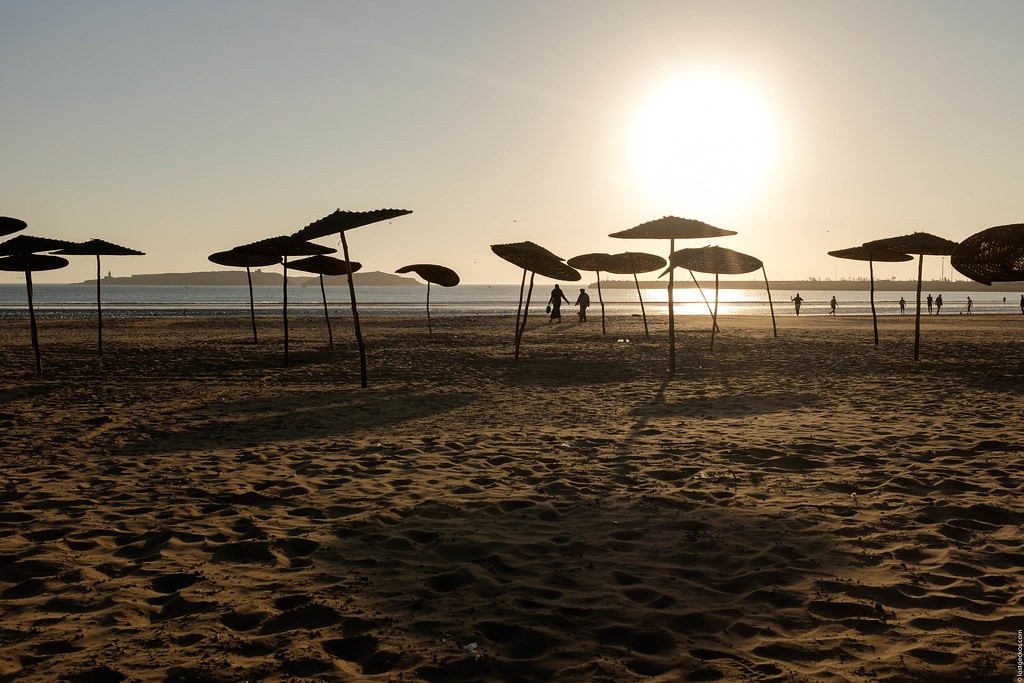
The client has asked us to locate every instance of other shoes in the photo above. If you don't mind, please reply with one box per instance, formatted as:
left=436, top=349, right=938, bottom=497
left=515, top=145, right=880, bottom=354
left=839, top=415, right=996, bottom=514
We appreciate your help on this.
left=583, top=321, right=586, bottom=322
left=548, top=320, right=551, bottom=322
left=557, top=321, right=561, bottom=323
left=578, top=319, right=581, bottom=322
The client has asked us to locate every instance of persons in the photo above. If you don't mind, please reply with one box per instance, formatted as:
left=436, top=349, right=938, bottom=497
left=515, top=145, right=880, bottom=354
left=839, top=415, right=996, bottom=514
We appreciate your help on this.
left=927, top=294, right=934, bottom=314
left=966, top=296, right=973, bottom=314
left=935, top=294, right=943, bottom=314
left=1020, top=295, right=1024, bottom=314
left=791, top=294, right=804, bottom=315
left=829, top=296, right=839, bottom=316
left=548, top=284, right=570, bottom=322
left=575, top=289, right=590, bottom=322
left=899, top=297, right=906, bottom=315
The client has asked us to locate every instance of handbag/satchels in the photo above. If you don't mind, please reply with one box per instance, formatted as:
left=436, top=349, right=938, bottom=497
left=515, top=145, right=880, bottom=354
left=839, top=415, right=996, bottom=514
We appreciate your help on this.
left=546, top=305, right=550, bottom=313
